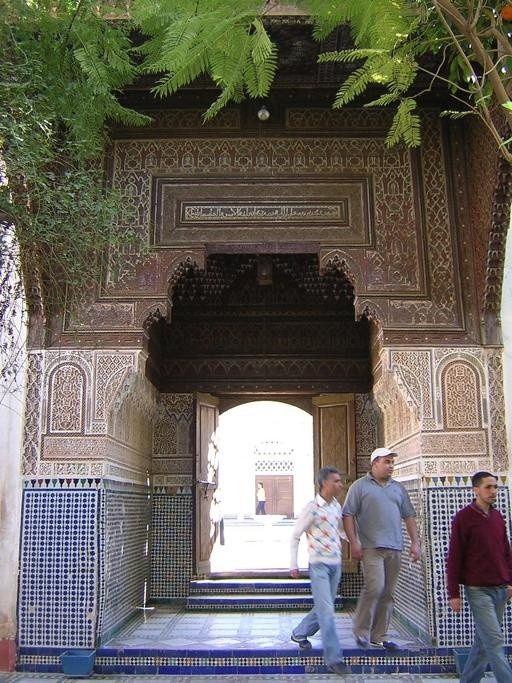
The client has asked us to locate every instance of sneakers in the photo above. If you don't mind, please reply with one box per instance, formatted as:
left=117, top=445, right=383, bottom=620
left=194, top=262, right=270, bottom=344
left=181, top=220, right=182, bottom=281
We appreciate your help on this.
left=326, top=661, right=352, bottom=674
left=368, top=638, right=400, bottom=651
left=352, top=629, right=371, bottom=649
left=290, top=634, right=312, bottom=649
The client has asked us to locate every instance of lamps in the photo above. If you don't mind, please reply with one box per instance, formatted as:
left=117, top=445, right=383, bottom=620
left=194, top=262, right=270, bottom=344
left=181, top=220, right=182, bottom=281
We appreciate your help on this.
left=256, top=253, right=274, bottom=285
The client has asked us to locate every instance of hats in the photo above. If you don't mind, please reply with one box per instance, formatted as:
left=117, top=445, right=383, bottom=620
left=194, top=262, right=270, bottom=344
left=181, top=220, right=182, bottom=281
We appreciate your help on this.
left=370, top=447, right=399, bottom=465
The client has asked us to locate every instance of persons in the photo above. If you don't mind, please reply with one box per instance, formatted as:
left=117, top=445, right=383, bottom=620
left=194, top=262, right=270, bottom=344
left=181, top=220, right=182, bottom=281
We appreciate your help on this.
left=342, top=446, right=422, bottom=649
left=445, top=470, right=512, bottom=683
left=254, top=481, right=268, bottom=514
left=209, top=484, right=226, bottom=547
left=289, top=465, right=362, bottom=678
